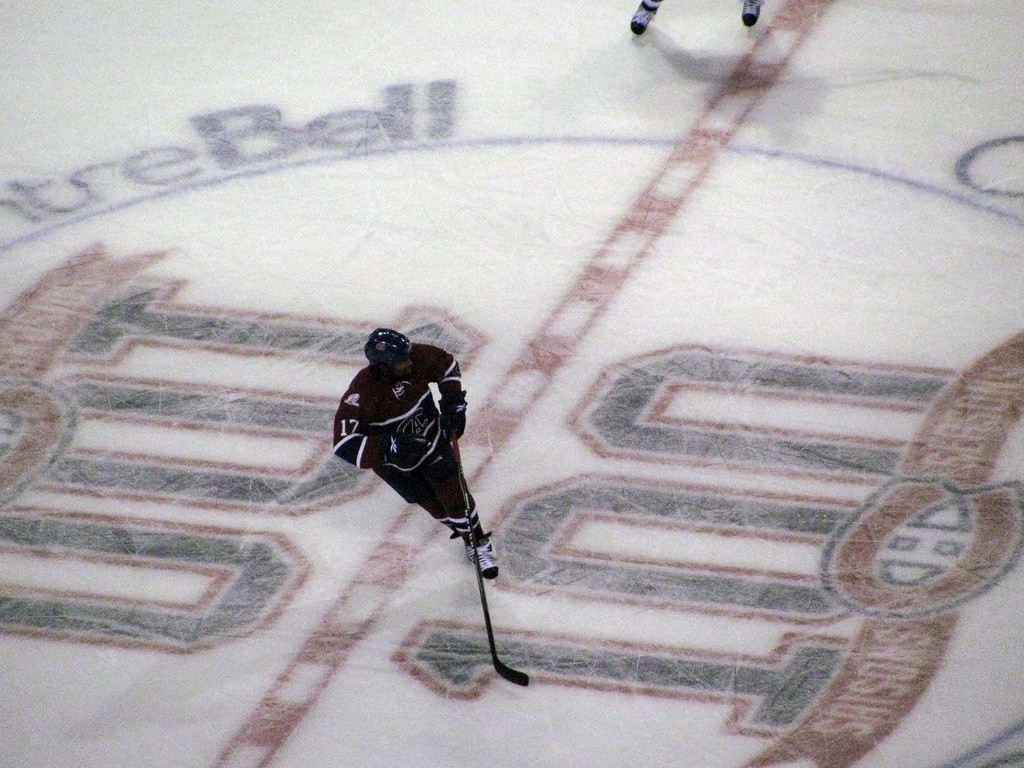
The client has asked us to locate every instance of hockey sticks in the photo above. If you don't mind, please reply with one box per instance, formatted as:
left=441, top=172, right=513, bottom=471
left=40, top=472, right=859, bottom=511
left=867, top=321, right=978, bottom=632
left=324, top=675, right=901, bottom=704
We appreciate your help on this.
left=447, top=431, right=531, bottom=688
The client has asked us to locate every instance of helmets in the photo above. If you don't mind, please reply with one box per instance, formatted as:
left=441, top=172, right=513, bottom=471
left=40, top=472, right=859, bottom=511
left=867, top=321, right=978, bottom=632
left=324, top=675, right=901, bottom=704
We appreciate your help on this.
left=364, top=328, right=410, bottom=371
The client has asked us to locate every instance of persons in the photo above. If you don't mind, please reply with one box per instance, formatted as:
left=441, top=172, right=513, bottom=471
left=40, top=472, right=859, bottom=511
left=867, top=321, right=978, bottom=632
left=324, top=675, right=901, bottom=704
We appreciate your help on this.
left=631, top=0, right=761, bottom=40
left=333, top=328, right=498, bottom=586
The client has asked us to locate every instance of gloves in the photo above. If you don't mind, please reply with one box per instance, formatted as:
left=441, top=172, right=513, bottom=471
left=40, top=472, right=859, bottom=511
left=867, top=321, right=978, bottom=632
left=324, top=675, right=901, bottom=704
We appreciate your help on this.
left=440, top=391, right=466, bottom=439
left=377, top=434, right=432, bottom=470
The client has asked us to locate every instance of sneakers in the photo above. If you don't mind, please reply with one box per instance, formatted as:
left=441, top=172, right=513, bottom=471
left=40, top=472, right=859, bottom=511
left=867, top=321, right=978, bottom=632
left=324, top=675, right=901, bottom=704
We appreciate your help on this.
left=630, top=0, right=663, bottom=35
left=742, top=0, right=764, bottom=27
left=465, top=531, right=498, bottom=579
